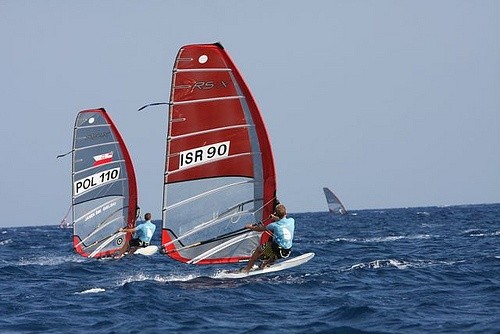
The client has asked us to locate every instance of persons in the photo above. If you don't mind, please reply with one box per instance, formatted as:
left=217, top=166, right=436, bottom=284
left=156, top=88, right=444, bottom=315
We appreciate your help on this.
left=118, top=212, right=156, bottom=253
left=240, top=204, right=295, bottom=272
left=338, top=207, right=343, bottom=215
